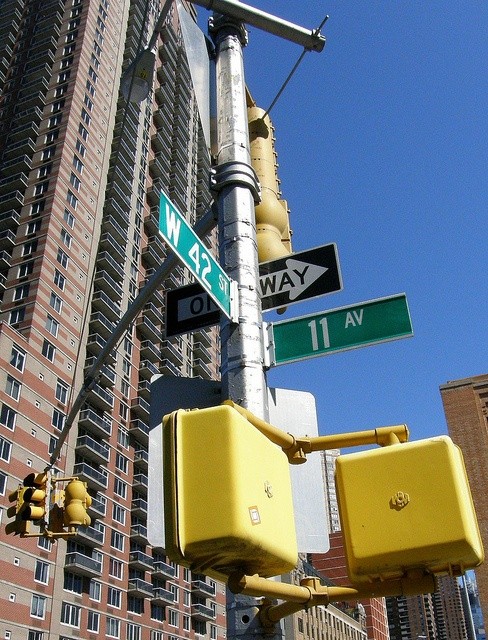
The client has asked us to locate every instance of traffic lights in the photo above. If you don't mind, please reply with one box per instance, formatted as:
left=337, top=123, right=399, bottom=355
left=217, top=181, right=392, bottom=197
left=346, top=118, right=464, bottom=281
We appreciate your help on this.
left=22, top=473, right=48, bottom=522
left=6, top=483, right=34, bottom=540
left=65, top=481, right=93, bottom=528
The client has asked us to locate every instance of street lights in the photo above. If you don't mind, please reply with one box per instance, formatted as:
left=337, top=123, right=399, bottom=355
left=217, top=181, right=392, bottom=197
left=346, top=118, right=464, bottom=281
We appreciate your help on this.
left=118, top=1, right=328, bottom=640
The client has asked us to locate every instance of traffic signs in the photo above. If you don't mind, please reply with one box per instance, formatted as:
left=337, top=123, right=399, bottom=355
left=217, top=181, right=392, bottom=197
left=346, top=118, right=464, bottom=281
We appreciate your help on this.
left=165, top=241, right=342, bottom=346
left=262, top=285, right=415, bottom=375
left=159, top=179, right=245, bottom=326
